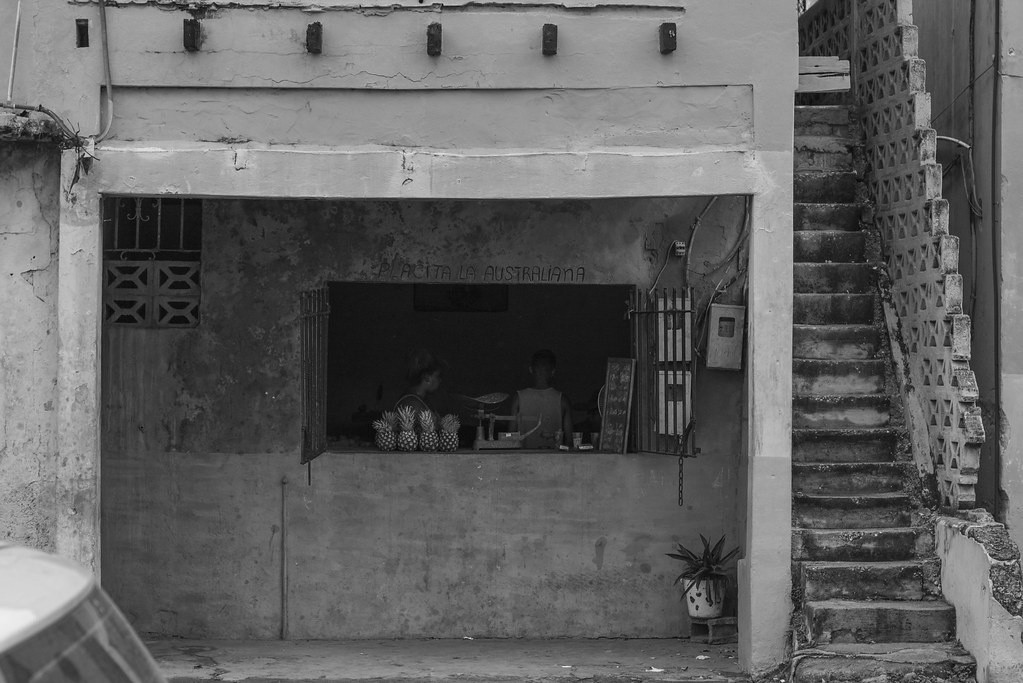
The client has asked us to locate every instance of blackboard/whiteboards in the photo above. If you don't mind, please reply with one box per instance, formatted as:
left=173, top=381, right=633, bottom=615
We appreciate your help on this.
left=599, top=357, right=636, bottom=453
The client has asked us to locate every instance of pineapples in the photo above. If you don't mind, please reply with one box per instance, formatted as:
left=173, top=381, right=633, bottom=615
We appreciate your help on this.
left=370, top=405, right=461, bottom=452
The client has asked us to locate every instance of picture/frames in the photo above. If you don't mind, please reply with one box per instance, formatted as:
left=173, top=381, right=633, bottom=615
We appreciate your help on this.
left=413, top=284, right=508, bottom=313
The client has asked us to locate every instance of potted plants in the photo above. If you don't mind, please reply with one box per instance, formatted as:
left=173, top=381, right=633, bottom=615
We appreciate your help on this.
left=662, top=531, right=741, bottom=620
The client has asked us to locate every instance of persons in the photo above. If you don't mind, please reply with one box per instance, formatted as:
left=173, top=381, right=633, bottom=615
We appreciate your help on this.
left=508, top=349, right=573, bottom=449
left=397, top=345, right=444, bottom=430
